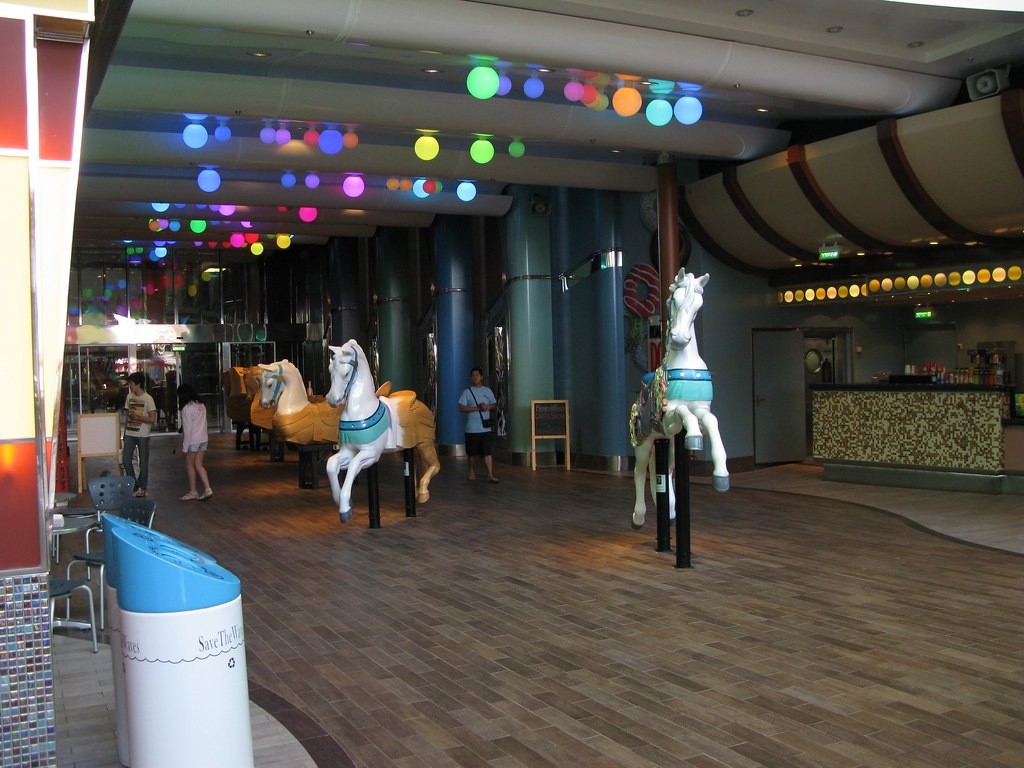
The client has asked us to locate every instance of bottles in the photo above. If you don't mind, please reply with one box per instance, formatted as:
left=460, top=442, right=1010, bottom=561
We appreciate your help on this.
left=904, top=361, right=931, bottom=375
left=932, top=362, right=973, bottom=384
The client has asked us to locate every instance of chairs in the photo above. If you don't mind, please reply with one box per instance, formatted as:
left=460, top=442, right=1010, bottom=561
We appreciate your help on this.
left=49, top=473, right=158, bottom=654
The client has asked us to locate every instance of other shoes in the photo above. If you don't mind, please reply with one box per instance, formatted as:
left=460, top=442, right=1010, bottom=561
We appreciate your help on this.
left=489, top=477, right=499, bottom=483
left=197, top=488, right=213, bottom=501
left=469, top=475, right=476, bottom=481
left=136, top=487, right=146, bottom=496
left=179, top=491, right=200, bottom=501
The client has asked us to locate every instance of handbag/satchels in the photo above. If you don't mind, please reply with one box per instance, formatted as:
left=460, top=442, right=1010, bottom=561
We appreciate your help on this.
left=482, top=418, right=495, bottom=428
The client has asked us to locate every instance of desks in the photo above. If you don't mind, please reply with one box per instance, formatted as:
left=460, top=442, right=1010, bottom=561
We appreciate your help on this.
left=54, top=491, right=77, bottom=508
left=51, top=517, right=95, bottom=632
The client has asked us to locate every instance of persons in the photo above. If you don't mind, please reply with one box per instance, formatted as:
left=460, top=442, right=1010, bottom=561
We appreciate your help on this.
left=177, top=383, right=213, bottom=501
left=122, top=372, right=157, bottom=496
left=458, top=368, right=500, bottom=483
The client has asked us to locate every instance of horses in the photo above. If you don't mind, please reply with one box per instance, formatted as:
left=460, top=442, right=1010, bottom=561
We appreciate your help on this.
left=239, top=366, right=276, bottom=429
left=629, top=267, right=729, bottom=531
left=258, top=359, right=339, bottom=444
left=325, top=339, right=441, bottom=524
